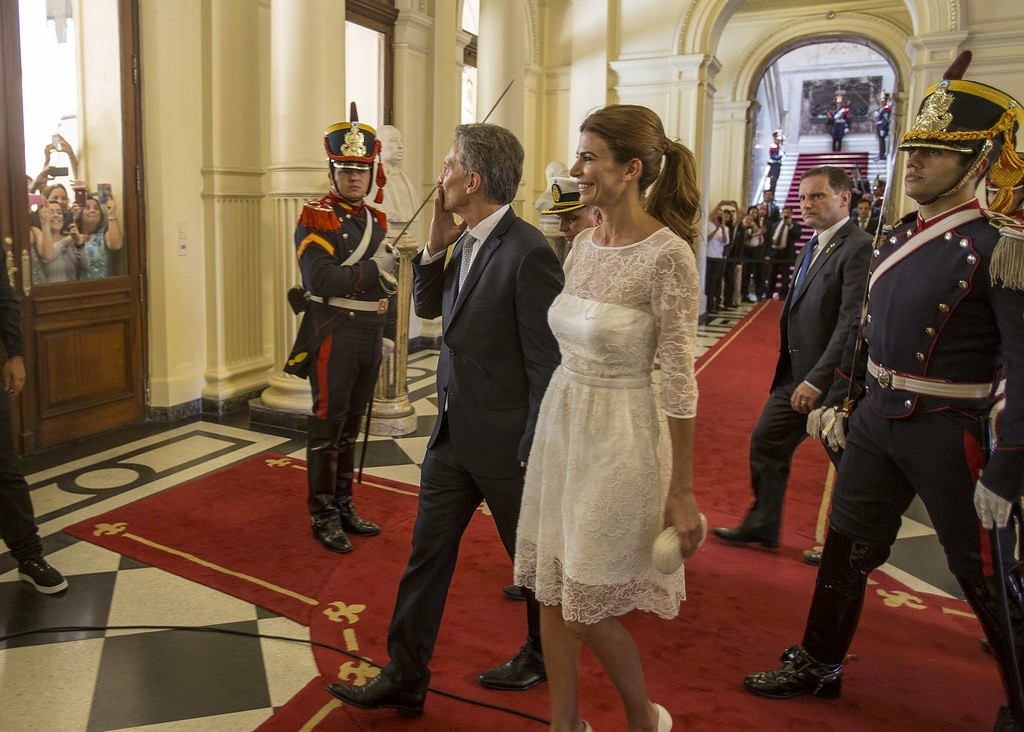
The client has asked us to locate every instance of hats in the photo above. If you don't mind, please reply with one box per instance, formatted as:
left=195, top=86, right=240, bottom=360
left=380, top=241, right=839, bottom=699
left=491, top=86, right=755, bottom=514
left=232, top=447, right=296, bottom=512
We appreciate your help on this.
left=899, top=50, right=1023, bottom=171
left=540, top=178, right=586, bottom=215
left=324, top=121, right=387, bottom=203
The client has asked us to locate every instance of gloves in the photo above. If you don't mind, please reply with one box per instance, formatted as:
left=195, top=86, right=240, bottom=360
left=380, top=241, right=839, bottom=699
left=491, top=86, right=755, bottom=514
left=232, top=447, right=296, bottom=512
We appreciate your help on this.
left=972, top=480, right=1012, bottom=528
left=373, top=238, right=401, bottom=276
left=821, top=408, right=847, bottom=452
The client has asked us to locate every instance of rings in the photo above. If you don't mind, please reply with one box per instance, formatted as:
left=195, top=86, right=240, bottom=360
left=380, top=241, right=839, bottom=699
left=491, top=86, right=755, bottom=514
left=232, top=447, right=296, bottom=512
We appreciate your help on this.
left=801, top=400, right=807, bottom=405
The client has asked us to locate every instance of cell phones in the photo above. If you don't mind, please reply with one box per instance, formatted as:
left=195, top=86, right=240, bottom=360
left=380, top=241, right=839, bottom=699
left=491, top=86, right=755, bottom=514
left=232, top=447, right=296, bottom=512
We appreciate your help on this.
left=62, top=212, right=73, bottom=229
left=74, top=187, right=86, bottom=208
left=52, top=135, right=58, bottom=147
left=97, top=184, right=113, bottom=204
left=50, top=167, right=68, bottom=176
left=27, top=195, right=46, bottom=205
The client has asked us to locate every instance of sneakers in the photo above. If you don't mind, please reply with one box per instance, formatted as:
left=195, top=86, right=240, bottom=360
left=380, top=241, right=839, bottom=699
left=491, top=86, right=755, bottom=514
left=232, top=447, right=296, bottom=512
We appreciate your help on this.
left=17, top=556, right=68, bottom=595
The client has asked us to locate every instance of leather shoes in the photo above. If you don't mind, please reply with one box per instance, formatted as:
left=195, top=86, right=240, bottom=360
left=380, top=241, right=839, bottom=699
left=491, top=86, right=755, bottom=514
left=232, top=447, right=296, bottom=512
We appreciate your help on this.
left=712, top=527, right=778, bottom=548
left=744, top=646, right=843, bottom=699
left=477, top=647, right=546, bottom=691
left=324, top=673, right=425, bottom=718
left=803, top=552, right=820, bottom=564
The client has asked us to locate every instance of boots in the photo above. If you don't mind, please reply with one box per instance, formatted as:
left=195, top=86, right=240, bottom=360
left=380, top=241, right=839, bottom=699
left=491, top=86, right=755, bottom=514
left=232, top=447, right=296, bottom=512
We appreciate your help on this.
left=336, top=414, right=382, bottom=536
left=307, top=414, right=352, bottom=553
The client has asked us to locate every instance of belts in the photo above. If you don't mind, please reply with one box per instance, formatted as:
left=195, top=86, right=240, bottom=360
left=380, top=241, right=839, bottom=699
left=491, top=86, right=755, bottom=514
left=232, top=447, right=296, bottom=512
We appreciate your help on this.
left=310, top=295, right=389, bottom=315
left=866, top=357, right=993, bottom=398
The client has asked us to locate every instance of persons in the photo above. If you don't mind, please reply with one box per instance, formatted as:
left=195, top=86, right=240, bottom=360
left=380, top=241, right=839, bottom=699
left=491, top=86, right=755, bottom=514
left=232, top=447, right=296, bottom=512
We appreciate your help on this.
left=711, top=165, right=875, bottom=567
left=282, top=101, right=399, bottom=551
left=534, top=160, right=570, bottom=211
left=365, top=124, right=422, bottom=218
left=765, top=128, right=786, bottom=203
left=513, top=105, right=704, bottom=732
left=325, top=122, right=567, bottom=720
left=0, top=238, right=70, bottom=593
left=503, top=176, right=604, bottom=601
left=704, top=189, right=801, bottom=316
left=25, top=135, right=123, bottom=285
left=735, top=52, right=1023, bottom=732
left=874, top=92, right=891, bottom=159
left=824, top=95, right=851, bottom=152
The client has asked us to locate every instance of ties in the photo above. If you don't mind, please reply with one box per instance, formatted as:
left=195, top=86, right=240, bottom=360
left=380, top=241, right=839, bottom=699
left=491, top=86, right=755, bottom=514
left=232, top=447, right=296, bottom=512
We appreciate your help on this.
left=861, top=220, right=866, bottom=230
left=776, top=223, right=786, bottom=245
left=458, top=234, right=477, bottom=293
left=790, top=236, right=819, bottom=308
left=766, top=205, right=769, bottom=217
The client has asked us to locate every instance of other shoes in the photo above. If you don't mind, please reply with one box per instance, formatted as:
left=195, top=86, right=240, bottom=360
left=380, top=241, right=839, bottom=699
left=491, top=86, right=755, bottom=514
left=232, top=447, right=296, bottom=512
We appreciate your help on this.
left=650, top=701, right=672, bottom=731
left=981, top=638, right=995, bottom=655
left=993, top=706, right=1023, bottom=732
left=581, top=719, right=593, bottom=732
left=504, top=584, right=527, bottom=601
left=706, top=285, right=788, bottom=314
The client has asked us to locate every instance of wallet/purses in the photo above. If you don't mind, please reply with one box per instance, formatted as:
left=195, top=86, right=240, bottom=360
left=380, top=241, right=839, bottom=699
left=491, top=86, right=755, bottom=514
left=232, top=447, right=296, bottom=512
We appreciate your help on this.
left=652, top=511, right=708, bottom=575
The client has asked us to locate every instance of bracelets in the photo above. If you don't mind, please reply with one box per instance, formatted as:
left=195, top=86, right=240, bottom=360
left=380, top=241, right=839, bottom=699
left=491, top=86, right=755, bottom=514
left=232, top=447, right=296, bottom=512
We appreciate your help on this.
left=75, top=240, right=84, bottom=248
left=108, top=217, right=118, bottom=221
